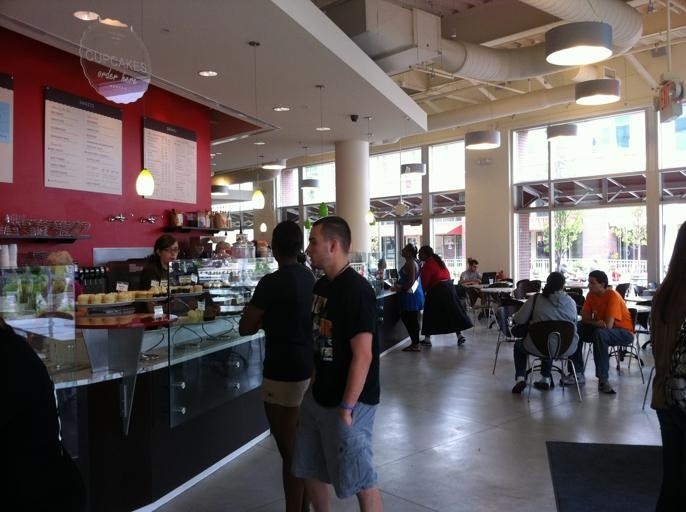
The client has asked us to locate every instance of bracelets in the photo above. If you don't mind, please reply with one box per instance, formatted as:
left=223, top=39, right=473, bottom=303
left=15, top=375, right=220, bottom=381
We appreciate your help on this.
left=338, top=402, right=357, bottom=412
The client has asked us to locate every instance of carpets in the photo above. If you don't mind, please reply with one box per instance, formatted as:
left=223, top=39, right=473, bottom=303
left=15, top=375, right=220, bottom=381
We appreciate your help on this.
left=546, top=440, right=665, bottom=511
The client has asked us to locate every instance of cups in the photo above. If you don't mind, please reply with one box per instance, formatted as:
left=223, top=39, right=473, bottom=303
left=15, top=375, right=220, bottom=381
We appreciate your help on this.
left=1, top=244, right=18, bottom=269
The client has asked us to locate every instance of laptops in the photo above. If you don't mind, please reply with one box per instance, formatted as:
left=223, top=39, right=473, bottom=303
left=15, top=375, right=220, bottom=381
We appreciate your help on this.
left=479, top=272, right=496, bottom=284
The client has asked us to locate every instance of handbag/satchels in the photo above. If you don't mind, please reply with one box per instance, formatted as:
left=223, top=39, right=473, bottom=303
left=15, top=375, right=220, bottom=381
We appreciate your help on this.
left=393, top=278, right=419, bottom=294
left=511, top=324, right=528, bottom=338
left=663, top=324, right=686, bottom=414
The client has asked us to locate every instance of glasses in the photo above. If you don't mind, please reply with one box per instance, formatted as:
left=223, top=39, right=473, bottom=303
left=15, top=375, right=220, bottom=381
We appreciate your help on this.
left=162, top=247, right=180, bottom=253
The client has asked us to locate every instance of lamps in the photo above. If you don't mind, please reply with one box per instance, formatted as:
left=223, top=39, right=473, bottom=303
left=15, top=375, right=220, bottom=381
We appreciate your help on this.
left=211, top=185, right=229, bottom=196
left=248, top=40, right=265, bottom=209
left=575, top=79, right=620, bottom=107
left=136, top=1, right=155, bottom=196
left=465, top=130, right=500, bottom=150
left=305, top=219, right=310, bottom=229
left=547, top=123, right=578, bottom=141
left=315, top=85, right=328, bottom=219
left=300, top=179, right=320, bottom=191
left=545, top=22, right=613, bottom=66
left=401, top=163, right=426, bottom=176
left=394, top=138, right=409, bottom=216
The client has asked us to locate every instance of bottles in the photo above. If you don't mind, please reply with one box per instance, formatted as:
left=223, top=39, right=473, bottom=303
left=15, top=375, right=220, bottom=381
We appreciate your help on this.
left=74, top=262, right=112, bottom=294
left=188, top=211, right=232, bottom=228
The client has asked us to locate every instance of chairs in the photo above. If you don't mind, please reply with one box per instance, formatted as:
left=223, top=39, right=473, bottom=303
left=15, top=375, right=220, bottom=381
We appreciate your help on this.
left=461, top=278, right=657, bottom=412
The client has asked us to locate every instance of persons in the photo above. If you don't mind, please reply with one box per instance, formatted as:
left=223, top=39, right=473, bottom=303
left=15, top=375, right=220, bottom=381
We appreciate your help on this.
left=1, top=317, right=59, bottom=511
left=460, top=259, right=486, bottom=308
left=513, top=271, right=579, bottom=393
left=391, top=244, right=425, bottom=352
left=135, top=234, right=180, bottom=313
left=239, top=221, right=314, bottom=511
left=651, top=220, right=685, bottom=511
left=27, top=250, right=86, bottom=347
left=419, top=245, right=473, bottom=346
left=293, top=217, right=384, bottom=511
left=561, top=271, right=634, bottom=394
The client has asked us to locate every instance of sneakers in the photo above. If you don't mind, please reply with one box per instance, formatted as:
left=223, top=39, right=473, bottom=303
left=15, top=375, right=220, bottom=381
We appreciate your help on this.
left=420, top=340, right=432, bottom=345
left=458, top=337, right=465, bottom=345
left=559, top=372, right=585, bottom=384
left=534, top=381, right=550, bottom=390
left=402, top=344, right=422, bottom=351
left=512, top=381, right=527, bottom=393
left=598, top=382, right=616, bottom=394
